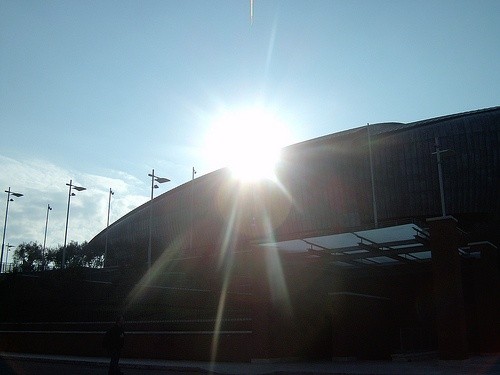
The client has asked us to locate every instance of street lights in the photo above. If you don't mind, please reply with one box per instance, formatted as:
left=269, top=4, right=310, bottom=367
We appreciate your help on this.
left=191, top=167, right=197, bottom=258
left=5, top=243, right=15, bottom=273
left=42, top=204, right=53, bottom=263
left=63, top=179, right=86, bottom=269
left=147, top=169, right=171, bottom=271
left=0, top=186, right=24, bottom=273
left=105, top=188, right=115, bottom=266
